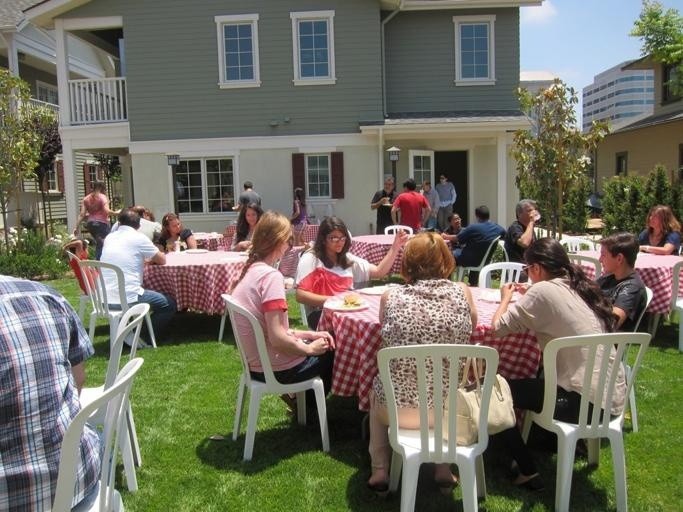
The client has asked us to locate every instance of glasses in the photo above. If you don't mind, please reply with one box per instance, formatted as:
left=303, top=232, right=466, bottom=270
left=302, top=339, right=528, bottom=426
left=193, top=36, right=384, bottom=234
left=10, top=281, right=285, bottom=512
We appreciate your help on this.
left=329, top=236, right=347, bottom=242
left=522, top=264, right=535, bottom=277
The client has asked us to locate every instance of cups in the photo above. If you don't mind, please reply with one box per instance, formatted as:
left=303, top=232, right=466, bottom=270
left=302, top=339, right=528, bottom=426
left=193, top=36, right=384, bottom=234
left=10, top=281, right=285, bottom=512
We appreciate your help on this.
left=384, top=197, right=390, bottom=204
left=420, top=190, right=425, bottom=195
left=531, top=210, right=540, bottom=221
left=174, top=241, right=180, bottom=254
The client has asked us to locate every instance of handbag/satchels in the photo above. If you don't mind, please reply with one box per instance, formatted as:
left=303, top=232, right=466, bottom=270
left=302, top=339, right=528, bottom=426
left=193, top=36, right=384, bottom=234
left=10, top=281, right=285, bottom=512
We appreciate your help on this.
left=441, top=374, right=517, bottom=446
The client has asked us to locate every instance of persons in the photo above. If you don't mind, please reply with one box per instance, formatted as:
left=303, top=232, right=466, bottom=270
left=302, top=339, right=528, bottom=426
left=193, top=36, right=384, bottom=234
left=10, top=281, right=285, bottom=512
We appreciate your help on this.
left=370, top=173, right=540, bottom=284
left=288, top=187, right=310, bottom=224
left=592, top=229, right=647, bottom=334
left=96, top=211, right=176, bottom=349
left=226, top=210, right=337, bottom=421
left=491, top=236, right=627, bottom=493
left=293, top=215, right=409, bottom=340
left=73, top=179, right=122, bottom=261
left=0, top=274, right=115, bottom=511
left=232, top=182, right=261, bottom=211
left=112, top=203, right=197, bottom=254
left=230, top=201, right=265, bottom=252
left=637, top=205, right=682, bottom=336
left=367, top=231, right=477, bottom=498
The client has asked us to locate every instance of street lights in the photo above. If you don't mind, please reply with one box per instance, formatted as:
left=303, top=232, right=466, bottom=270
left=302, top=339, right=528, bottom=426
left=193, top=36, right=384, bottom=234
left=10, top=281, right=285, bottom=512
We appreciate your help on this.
left=164, top=151, right=181, bottom=219
left=386, top=146, right=401, bottom=223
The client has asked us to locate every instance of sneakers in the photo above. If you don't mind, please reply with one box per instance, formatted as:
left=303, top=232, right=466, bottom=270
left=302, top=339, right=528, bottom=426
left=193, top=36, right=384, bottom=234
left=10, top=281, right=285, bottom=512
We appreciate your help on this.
left=516, top=472, right=547, bottom=493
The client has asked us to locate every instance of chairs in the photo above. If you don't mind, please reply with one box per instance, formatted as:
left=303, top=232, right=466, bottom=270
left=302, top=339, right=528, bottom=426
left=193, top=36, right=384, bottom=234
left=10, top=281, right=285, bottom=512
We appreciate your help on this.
left=69, top=301, right=152, bottom=493
left=369, top=338, right=501, bottom=510
left=517, top=329, right=654, bottom=507
left=43, top=355, right=128, bottom=511
left=217, top=291, right=331, bottom=463
left=24, top=214, right=679, bottom=353
left=620, top=283, right=652, bottom=436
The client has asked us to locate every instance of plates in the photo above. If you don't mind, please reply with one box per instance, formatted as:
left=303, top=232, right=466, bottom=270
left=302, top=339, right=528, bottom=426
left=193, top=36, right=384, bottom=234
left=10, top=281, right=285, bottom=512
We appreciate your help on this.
left=382, top=204, right=393, bottom=206
left=321, top=299, right=370, bottom=311
left=478, top=290, right=518, bottom=303
left=360, top=285, right=388, bottom=296
left=185, top=248, right=208, bottom=253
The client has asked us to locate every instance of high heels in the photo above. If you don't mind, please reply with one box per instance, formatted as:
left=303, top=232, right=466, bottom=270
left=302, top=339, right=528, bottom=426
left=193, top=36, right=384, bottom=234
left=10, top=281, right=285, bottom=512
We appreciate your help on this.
left=437, top=472, right=458, bottom=496
left=367, top=479, right=390, bottom=498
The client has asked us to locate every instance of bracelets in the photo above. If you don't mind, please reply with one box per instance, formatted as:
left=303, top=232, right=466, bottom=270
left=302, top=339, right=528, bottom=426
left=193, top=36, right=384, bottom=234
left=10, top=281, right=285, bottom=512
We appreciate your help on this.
left=646, top=244, right=650, bottom=252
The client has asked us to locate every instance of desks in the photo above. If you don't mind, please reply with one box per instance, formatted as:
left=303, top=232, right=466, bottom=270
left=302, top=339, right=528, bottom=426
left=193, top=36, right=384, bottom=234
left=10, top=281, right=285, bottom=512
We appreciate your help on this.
left=320, top=276, right=542, bottom=453
